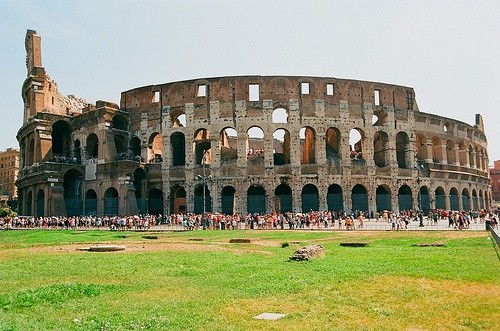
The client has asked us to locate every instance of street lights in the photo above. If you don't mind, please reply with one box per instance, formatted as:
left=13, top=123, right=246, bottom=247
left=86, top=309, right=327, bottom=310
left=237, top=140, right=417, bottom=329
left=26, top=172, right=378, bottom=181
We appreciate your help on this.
left=418, top=164, right=424, bottom=215
left=202, top=149, right=211, bottom=217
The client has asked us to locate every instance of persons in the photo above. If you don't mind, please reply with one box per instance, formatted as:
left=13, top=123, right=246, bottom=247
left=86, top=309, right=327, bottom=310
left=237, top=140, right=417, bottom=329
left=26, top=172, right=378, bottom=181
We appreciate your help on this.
left=0, top=149, right=498, bottom=235
